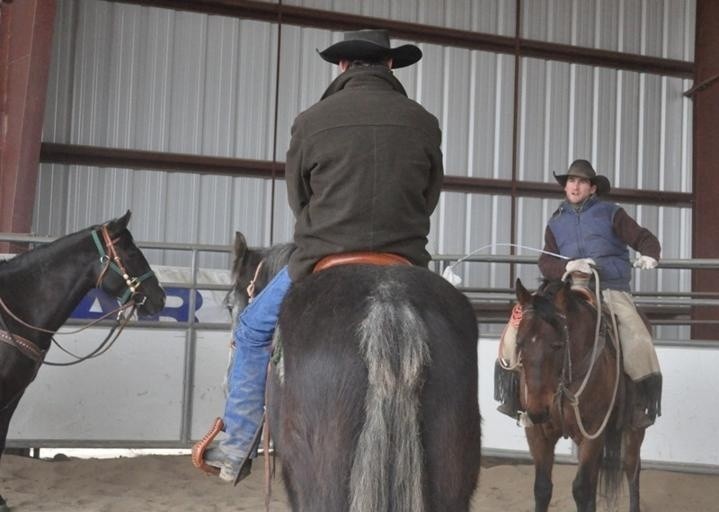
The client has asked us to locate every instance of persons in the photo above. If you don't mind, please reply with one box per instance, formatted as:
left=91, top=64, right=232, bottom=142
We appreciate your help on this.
left=493, top=158, right=661, bottom=426
left=197, top=28, right=444, bottom=484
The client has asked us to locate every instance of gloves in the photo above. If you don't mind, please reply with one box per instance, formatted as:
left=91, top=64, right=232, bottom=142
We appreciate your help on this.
left=634, top=256, right=657, bottom=271
left=566, top=258, right=594, bottom=277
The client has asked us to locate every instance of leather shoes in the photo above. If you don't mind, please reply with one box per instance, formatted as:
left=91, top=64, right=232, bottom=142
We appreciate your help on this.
left=633, top=413, right=652, bottom=431
left=203, top=447, right=250, bottom=483
left=497, top=403, right=514, bottom=414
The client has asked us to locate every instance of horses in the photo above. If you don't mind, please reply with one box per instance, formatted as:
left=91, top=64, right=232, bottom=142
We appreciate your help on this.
left=220, top=229, right=484, bottom=512
left=1, top=206, right=168, bottom=512
left=513, top=277, right=646, bottom=512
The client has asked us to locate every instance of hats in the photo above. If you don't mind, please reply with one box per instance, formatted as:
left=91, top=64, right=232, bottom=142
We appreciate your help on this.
left=316, top=30, right=422, bottom=69
left=553, top=160, right=610, bottom=195
